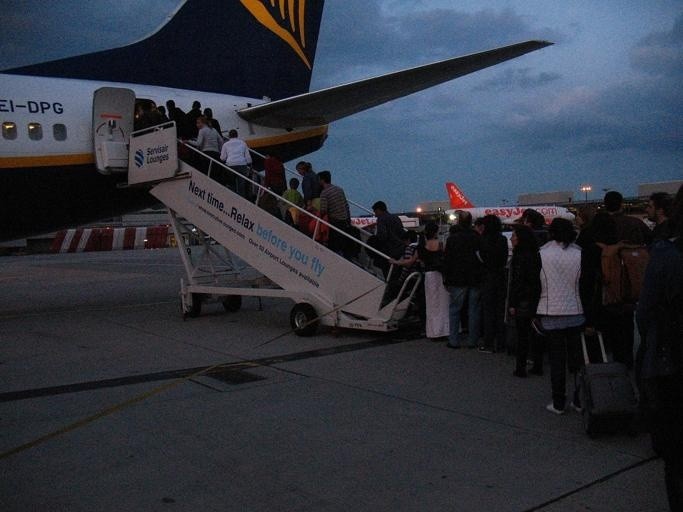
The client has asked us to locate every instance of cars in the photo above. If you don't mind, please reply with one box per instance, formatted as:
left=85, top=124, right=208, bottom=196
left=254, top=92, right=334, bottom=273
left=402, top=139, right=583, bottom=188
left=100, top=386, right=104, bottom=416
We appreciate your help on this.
left=173, top=216, right=199, bottom=241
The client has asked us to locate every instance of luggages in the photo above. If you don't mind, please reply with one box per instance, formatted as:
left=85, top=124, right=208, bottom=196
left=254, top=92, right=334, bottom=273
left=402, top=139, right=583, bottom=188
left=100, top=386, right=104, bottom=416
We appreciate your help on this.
left=580, top=331, right=640, bottom=430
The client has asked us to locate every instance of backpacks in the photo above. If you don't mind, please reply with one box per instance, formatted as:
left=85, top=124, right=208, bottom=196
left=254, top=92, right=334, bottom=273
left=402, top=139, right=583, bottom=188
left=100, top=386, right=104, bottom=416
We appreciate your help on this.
left=596, top=239, right=648, bottom=306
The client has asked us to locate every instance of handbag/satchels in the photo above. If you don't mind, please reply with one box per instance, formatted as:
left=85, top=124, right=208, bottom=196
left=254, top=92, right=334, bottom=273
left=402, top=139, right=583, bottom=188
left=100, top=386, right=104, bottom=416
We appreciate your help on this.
left=349, top=226, right=361, bottom=252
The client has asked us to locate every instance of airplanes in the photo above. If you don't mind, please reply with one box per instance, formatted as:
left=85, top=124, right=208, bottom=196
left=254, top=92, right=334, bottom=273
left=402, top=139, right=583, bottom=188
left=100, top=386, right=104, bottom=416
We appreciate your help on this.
left=441, top=181, right=579, bottom=229
left=0, top=1, right=555, bottom=244
left=351, top=214, right=420, bottom=232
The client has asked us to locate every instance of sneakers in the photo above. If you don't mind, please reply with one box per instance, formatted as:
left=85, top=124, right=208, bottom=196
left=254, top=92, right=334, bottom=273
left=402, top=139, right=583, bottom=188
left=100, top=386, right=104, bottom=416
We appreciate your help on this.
left=545, top=404, right=563, bottom=414
left=528, top=368, right=544, bottom=376
left=477, top=344, right=494, bottom=351
left=513, top=368, right=526, bottom=377
left=570, top=401, right=583, bottom=412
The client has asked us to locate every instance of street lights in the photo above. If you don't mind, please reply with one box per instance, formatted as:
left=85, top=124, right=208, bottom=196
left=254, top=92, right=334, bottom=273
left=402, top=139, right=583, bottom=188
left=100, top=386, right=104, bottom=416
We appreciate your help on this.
left=582, top=185, right=591, bottom=201
